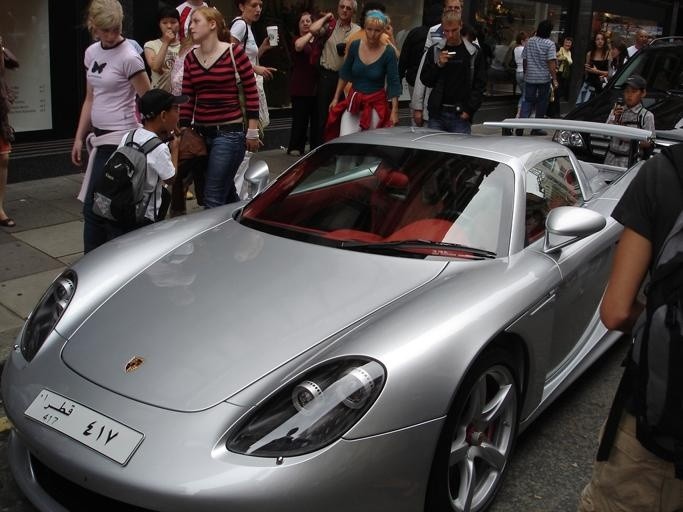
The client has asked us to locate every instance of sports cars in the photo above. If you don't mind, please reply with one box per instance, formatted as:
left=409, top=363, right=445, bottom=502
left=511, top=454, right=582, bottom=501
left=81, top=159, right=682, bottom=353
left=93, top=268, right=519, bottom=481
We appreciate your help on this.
left=1, top=118, right=682, bottom=511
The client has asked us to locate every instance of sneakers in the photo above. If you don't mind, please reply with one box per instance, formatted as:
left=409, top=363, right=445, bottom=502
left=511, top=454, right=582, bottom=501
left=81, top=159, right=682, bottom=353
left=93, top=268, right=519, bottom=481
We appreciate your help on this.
left=290, top=150, right=301, bottom=157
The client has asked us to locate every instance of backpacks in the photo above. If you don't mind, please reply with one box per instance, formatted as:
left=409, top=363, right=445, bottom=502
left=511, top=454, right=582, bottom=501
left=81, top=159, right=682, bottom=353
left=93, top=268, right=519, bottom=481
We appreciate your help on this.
left=88, top=128, right=165, bottom=230
left=595, top=209, right=683, bottom=482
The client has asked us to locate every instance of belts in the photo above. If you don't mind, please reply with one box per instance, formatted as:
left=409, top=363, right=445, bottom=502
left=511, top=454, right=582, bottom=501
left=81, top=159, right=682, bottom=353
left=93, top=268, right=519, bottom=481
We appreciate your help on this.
left=195, top=123, right=242, bottom=133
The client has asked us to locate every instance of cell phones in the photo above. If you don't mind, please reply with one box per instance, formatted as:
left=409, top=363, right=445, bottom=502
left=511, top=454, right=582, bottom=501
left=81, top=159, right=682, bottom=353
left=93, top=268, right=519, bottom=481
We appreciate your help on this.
left=617, top=94, right=625, bottom=111
left=448, top=52, right=456, bottom=55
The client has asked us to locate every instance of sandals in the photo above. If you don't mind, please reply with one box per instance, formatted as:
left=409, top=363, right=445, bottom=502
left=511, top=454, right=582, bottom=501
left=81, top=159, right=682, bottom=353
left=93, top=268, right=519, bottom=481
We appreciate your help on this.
left=1, top=216, right=14, bottom=227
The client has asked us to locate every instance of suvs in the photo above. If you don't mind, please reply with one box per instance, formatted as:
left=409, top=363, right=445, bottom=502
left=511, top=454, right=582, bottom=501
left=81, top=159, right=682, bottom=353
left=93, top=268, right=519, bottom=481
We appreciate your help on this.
left=551, top=35, right=682, bottom=164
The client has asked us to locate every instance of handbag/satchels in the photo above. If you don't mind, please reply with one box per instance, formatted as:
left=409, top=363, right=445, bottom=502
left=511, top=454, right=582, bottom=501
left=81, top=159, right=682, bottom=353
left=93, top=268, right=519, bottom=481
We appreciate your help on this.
left=310, top=18, right=337, bottom=68
left=230, top=43, right=264, bottom=143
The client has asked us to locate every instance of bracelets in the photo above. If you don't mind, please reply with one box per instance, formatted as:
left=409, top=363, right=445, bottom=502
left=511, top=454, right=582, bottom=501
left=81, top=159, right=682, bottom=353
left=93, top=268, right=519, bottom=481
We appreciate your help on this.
left=1, top=46, right=4, bottom=52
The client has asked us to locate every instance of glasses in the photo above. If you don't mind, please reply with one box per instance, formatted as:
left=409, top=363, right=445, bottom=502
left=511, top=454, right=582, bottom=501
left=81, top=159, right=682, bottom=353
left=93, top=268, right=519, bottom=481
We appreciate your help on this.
left=340, top=4, right=353, bottom=12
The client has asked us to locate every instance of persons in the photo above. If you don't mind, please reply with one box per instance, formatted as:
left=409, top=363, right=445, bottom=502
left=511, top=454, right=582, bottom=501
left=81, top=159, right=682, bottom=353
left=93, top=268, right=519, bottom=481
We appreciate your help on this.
left=556, top=36, right=572, bottom=79
left=397, top=11, right=440, bottom=127
left=71, top=0, right=152, bottom=255
left=513, top=31, right=529, bottom=118
left=175, top=0, right=209, bottom=45
left=227, top=0, right=279, bottom=202
left=489, top=34, right=510, bottom=95
left=308, top=0, right=361, bottom=151
left=120, top=20, right=152, bottom=84
left=576, top=143, right=683, bottom=512
left=142, top=7, right=193, bottom=219
left=323, top=9, right=402, bottom=176
left=177, top=6, right=260, bottom=211
left=0, top=36, right=20, bottom=227
left=574, top=30, right=609, bottom=109
left=602, top=74, right=655, bottom=168
left=419, top=10, right=488, bottom=134
left=408, top=23, right=477, bottom=128
left=601, top=41, right=629, bottom=80
left=514, top=20, right=559, bottom=136
left=624, top=29, right=647, bottom=64
left=423, top=0, right=480, bottom=52
left=115, top=88, right=181, bottom=236
left=287, top=11, right=317, bottom=155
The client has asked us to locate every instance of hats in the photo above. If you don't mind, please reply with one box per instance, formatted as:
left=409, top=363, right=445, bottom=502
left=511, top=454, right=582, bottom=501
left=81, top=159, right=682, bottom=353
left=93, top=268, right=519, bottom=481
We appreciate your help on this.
left=621, top=75, right=647, bottom=91
left=138, top=89, right=189, bottom=120
